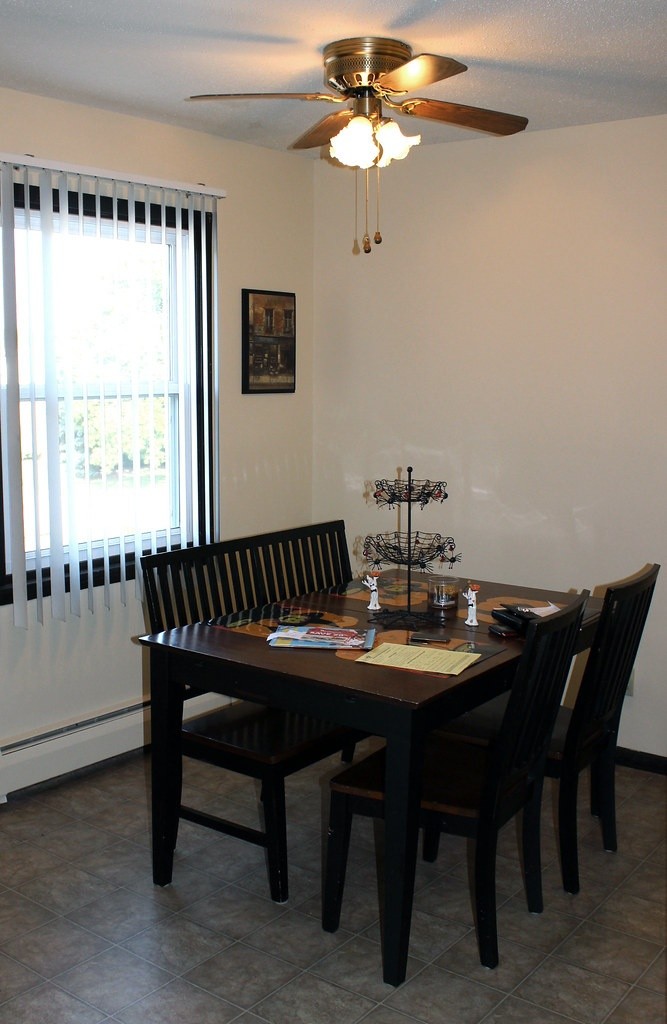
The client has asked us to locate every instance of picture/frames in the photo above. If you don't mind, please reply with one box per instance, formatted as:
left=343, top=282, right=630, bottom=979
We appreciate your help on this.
left=241, top=288, right=296, bottom=394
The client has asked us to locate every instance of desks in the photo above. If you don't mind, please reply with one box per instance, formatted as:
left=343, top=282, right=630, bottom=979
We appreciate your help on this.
left=138, top=568, right=617, bottom=989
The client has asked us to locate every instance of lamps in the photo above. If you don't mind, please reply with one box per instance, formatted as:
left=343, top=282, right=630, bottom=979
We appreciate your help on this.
left=329, top=96, right=422, bottom=169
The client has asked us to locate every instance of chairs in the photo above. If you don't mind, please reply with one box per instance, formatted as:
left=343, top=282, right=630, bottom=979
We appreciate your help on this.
left=140, top=520, right=661, bottom=987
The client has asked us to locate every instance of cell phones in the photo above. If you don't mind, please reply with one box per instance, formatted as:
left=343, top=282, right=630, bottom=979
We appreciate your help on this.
left=488, top=604, right=540, bottom=637
left=410, top=634, right=449, bottom=643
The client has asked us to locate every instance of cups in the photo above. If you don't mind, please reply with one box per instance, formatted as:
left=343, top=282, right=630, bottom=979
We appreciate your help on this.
left=429, top=575, right=459, bottom=609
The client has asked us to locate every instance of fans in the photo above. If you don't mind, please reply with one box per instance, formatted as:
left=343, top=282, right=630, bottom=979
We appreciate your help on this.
left=190, top=37, right=529, bottom=150
